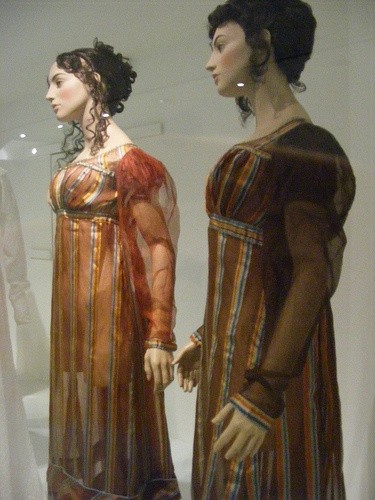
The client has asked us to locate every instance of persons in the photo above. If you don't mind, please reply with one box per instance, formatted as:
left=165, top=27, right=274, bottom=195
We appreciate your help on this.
left=38, top=41, right=184, bottom=498
left=168, top=0, right=360, bottom=499
